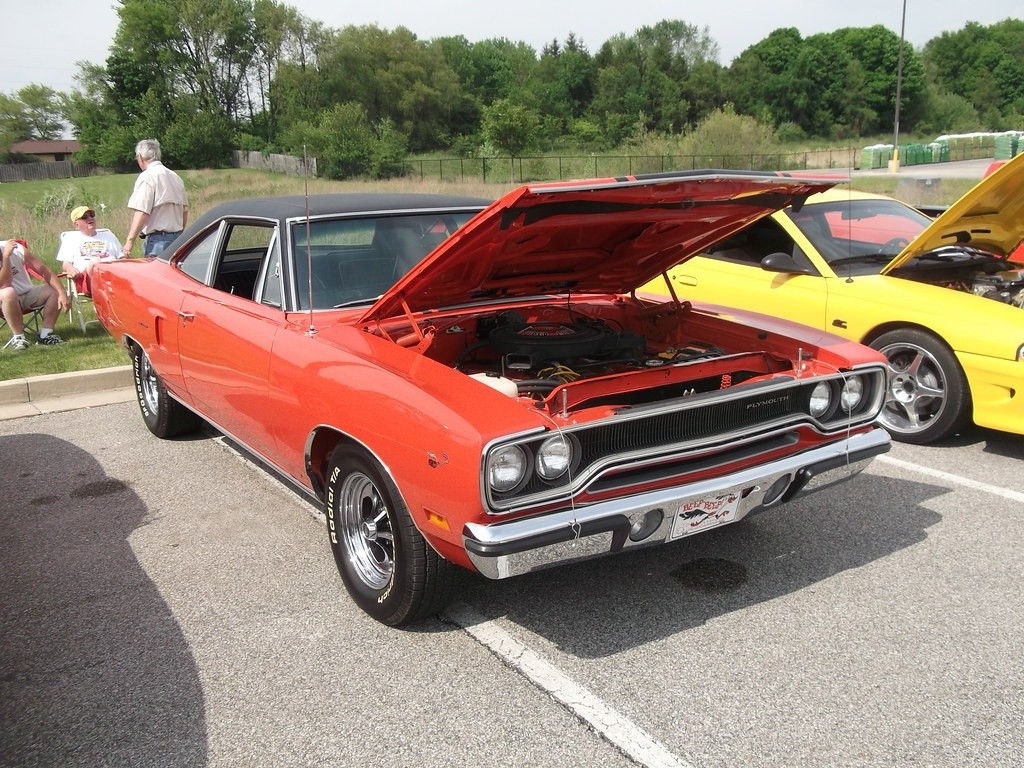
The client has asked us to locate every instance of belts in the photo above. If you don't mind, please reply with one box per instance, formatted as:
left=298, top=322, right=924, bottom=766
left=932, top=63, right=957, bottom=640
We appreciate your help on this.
left=150, top=230, right=179, bottom=234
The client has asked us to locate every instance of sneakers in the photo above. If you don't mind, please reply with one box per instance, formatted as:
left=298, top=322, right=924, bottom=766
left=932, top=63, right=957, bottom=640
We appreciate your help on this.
left=35, top=332, right=64, bottom=345
left=12, top=334, right=30, bottom=350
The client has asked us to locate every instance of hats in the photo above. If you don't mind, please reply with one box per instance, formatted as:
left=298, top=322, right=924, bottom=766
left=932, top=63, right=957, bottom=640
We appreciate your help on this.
left=70, top=206, right=96, bottom=223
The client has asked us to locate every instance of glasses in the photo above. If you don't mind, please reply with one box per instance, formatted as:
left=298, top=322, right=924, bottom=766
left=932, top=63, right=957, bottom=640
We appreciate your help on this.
left=81, top=213, right=95, bottom=219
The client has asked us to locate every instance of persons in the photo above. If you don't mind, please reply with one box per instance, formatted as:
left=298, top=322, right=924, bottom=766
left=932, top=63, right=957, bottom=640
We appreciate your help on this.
left=56, top=205, right=127, bottom=297
left=0, top=239, right=72, bottom=349
left=124, top=140, right=189, bottom=258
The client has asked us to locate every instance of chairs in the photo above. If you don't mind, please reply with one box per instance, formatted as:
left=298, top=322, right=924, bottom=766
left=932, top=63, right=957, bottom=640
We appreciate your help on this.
left=793, top=222, right=825, bottom=270
left=251, top=248, right=349, bottom=309
left=0, top=240, right=47, bottom=350
left=58, top=228, right=110, bottom=335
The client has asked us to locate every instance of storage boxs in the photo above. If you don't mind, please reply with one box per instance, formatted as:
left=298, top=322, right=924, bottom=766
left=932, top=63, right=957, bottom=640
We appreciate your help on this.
left=857, top=130, right=1024, bottom=169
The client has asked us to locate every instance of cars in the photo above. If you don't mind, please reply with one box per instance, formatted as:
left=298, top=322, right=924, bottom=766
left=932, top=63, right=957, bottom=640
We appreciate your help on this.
left=635, top=151, right=1024, bottom=447
left=89, top=169, right=890, bottom=629
